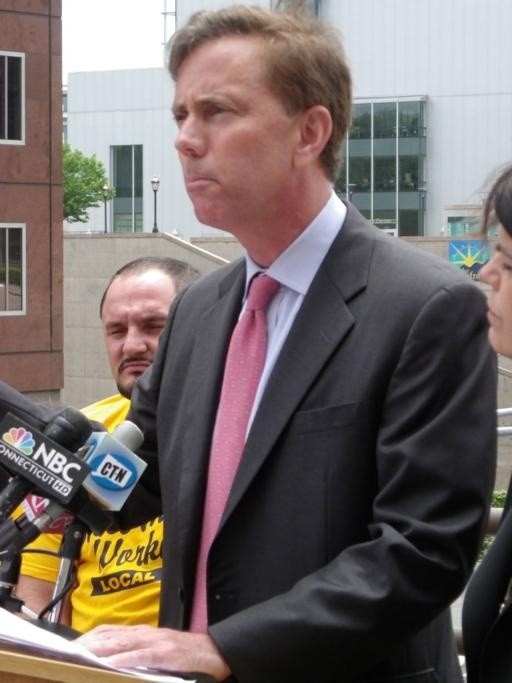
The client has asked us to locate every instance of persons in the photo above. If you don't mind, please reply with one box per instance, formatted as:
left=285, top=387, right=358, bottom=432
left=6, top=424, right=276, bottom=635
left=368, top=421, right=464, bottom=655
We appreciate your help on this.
left=5, top=254, right=202, bottom=639
left=72, top=1, right=498, bottom=683
left=458, top=159, right=512, bottom=682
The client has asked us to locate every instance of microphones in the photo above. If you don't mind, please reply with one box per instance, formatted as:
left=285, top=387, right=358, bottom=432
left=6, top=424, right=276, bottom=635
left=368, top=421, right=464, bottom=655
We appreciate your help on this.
left=0, top=420, right=148, bottom=560
left=0, top=406, right=93, bottom=526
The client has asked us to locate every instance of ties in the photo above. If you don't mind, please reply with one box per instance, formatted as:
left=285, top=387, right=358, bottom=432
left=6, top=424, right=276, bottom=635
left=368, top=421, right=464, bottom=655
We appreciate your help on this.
left=187, top=276, right=280, bottom=633
